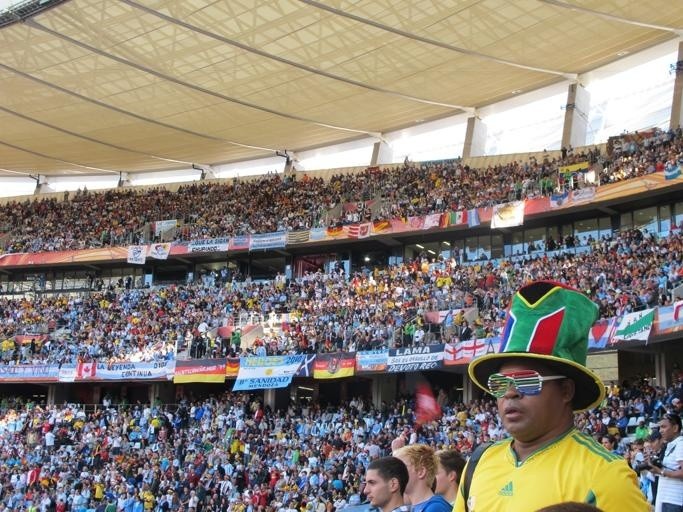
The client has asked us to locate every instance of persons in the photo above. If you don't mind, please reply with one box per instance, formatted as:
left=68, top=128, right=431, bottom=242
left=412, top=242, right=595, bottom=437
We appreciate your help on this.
left=0, top=126, right=683, bottom=512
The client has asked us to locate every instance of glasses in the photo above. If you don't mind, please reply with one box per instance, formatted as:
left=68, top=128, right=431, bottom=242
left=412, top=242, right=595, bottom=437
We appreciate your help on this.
left=487, top=370, right=563, bottom=400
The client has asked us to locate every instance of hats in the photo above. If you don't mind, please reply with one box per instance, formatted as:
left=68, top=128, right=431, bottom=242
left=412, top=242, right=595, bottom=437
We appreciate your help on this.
left=466, top=279, right=606, bottom=414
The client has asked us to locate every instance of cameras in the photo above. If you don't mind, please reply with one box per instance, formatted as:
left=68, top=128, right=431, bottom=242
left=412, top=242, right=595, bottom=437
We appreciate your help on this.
left=636, top=459, right=661, bottom=473
